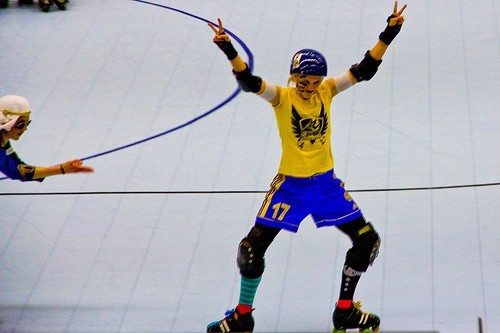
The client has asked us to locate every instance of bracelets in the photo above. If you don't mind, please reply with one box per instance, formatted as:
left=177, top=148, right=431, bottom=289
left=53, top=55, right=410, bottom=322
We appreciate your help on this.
left=60, top=165, right=65, bottom=174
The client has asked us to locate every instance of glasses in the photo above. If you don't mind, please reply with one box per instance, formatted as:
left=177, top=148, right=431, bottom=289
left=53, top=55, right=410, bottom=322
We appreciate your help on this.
left=16, top=120, right=32, bottom=129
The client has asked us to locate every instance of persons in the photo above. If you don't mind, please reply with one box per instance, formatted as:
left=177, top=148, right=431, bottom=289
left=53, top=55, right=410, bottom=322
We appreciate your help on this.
left=0, top=95, right=95, bottom=183
left=207, top=1, right=407, bottom=333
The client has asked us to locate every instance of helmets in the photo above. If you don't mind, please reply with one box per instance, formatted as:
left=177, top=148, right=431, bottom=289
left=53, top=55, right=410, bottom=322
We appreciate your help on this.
left=290, top=49, right=328, bottom=78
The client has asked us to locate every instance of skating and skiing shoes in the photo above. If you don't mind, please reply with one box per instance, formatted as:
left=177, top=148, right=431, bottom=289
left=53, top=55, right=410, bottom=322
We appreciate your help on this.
left=205, top=308, right=255, bottom=333
left=331, top=302, right=381, bottom=333
